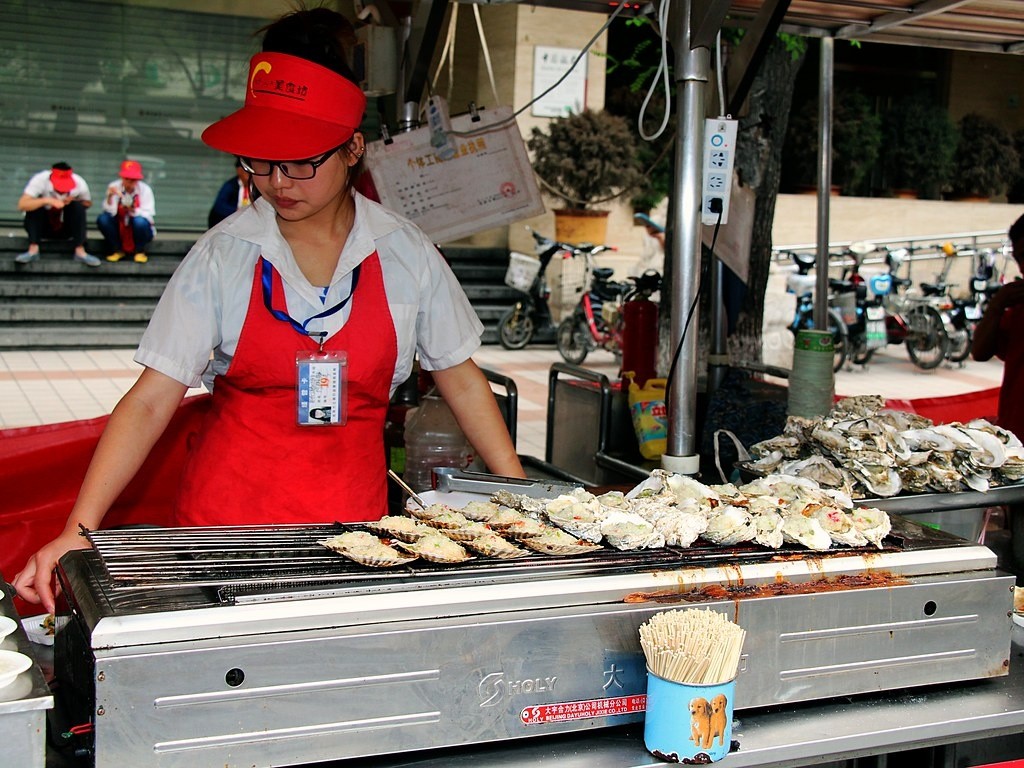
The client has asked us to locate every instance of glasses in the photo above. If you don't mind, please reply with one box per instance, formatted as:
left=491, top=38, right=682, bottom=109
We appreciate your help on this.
left=238, top=143, right=348, bottom=180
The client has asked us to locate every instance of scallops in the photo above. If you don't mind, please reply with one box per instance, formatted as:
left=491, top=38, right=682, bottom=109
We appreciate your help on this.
left=317, top=530, right=381, bottom=553
left=335, top=543, right=423, bottom=568
left=379, top=502, right=604, bottom=565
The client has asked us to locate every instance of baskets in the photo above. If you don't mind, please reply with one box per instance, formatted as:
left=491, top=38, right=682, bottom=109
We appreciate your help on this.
left=504, top=252, right=542, bottom=293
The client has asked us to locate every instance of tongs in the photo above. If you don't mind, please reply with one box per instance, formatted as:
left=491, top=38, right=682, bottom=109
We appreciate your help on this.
left=430, top=465, right=585, bottom=501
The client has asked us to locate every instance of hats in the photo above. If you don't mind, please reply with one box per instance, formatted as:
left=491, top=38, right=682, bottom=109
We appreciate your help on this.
left=119, top=161, right=144, bottom=180
left=51, top=168, right=76, bottom=193
left=199, top=53, right=367, bottom=161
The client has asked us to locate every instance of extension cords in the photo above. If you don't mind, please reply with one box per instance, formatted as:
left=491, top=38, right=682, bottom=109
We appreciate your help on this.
left=423, top=95, right=457, bottom=162
left=702, top=118, right=738, bottom=225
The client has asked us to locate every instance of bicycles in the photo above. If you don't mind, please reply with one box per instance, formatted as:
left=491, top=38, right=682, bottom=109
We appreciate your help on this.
left=551, top=243, right=664, bottom=366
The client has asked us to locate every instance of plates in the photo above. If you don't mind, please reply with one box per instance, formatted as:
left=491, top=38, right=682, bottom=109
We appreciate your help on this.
left=406, top=489, right=508, bottom=511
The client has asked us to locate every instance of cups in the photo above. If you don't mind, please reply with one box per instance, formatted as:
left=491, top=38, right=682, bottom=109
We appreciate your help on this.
left=643, top=662, right=739, bottom=765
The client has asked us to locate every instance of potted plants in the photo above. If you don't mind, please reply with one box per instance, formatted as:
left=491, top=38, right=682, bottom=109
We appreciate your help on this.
left=528, top=107, right=636, bottom=244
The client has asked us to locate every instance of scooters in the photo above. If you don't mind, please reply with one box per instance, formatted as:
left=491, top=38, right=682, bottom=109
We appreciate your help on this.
left=771, top=238, right=1017, bottom=374
left=496, top=224, right=635, bottom=352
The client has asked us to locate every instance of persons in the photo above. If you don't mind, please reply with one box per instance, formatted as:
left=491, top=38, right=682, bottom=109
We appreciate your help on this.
left=310, top=406, right=331, bottom=422
left=14, top=162, right=101, bottom=267
left=10, top=8, right=527, bottom=615
left=971, top=214, right=1024, bottom=584
left=207, top=157, right=255, bottom=230
left=96, top=161, right=157, bottom=263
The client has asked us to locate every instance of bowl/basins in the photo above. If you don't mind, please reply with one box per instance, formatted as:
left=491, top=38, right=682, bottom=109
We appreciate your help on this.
left=784, top=328, right=836, bottom=422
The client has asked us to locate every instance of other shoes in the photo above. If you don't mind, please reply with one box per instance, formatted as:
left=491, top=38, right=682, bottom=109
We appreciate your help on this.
left=135, top=254, right=148, bottom=263
left=107, top=253, right=127, bottom=261
left=74, top=253, right=101, bottom=266
left=15, top=253, right=40, bottom=263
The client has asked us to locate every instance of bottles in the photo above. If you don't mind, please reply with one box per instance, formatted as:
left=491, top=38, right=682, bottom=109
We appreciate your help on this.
left=384, top=360, right=436, bottom=487
left=403, top=381, right=467, bottom=491
